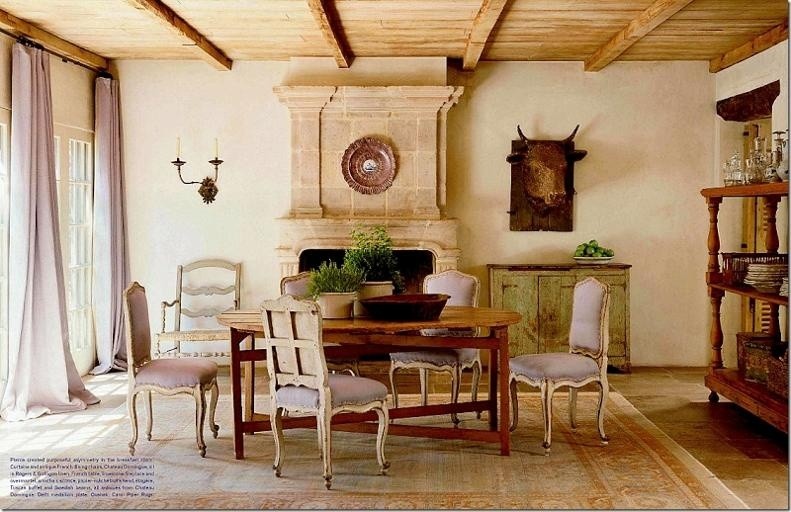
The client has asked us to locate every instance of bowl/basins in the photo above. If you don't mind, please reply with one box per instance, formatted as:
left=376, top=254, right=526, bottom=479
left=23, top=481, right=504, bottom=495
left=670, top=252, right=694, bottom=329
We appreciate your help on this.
left=573, top=255, right=613, bottom=268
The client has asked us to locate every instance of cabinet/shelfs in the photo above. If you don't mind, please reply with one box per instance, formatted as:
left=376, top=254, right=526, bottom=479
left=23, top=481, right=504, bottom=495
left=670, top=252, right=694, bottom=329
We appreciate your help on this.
left=487, top=262, right=634, bottom=375
left=702, top=180, right=789, bottom=434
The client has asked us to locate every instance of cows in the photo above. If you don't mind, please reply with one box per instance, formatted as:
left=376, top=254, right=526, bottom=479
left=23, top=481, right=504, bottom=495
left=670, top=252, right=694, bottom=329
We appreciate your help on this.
left=505, top=125, right=587, bottom=218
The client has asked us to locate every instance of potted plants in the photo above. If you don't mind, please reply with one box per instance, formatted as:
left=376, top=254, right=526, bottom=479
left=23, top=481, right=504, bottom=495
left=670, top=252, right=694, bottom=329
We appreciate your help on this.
left=307, top=224, right=400, bottom=319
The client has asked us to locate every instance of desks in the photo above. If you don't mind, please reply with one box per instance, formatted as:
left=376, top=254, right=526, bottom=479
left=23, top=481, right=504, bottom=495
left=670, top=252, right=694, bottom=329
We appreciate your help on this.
left=217, top=307, right=524, bottom=461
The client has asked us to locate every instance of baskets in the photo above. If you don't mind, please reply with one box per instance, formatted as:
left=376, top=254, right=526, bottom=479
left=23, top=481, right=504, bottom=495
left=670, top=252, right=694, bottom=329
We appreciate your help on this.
left=765, top=354, right=789, bottom=399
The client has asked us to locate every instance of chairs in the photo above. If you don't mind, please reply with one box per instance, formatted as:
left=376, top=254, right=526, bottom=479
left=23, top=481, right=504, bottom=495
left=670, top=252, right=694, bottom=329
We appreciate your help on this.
left=261, top=270, right=484, bottom=492
left=124, top=259, right=244, bottom=458
left=509, top=274, right=612, bottom=456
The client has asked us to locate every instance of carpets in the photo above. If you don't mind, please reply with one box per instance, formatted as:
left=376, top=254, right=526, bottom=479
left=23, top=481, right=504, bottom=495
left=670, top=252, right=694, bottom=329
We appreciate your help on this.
left=691, top=401, right=789, bottom=461
left=3, top=391, right=749, bottom=509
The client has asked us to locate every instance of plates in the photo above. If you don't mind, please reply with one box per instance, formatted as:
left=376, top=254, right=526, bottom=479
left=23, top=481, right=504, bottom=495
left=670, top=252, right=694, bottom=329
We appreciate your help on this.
left=742, top=263, right=788, bottom=293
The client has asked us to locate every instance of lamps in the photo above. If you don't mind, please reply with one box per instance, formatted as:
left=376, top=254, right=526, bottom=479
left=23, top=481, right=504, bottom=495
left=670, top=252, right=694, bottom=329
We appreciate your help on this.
left=171, top=137, right=224, bottom=205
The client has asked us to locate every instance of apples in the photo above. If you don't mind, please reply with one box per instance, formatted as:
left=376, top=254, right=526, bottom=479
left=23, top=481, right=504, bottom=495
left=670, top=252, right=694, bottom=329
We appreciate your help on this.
left=575, top=240, right=614, bottom=257
